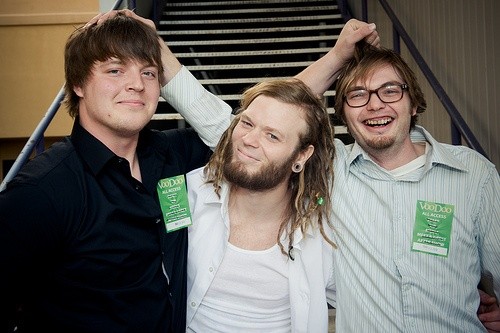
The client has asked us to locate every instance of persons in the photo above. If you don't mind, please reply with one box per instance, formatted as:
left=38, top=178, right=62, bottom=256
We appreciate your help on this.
left=83, top=9, right=500, bottom=333
left=0, top=18, right=381, bottom=333
left=186, top=78, right=500, bottom=333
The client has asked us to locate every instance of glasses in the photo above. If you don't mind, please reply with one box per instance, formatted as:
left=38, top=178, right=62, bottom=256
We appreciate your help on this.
left=344, top=83, right=408, bottom=108
